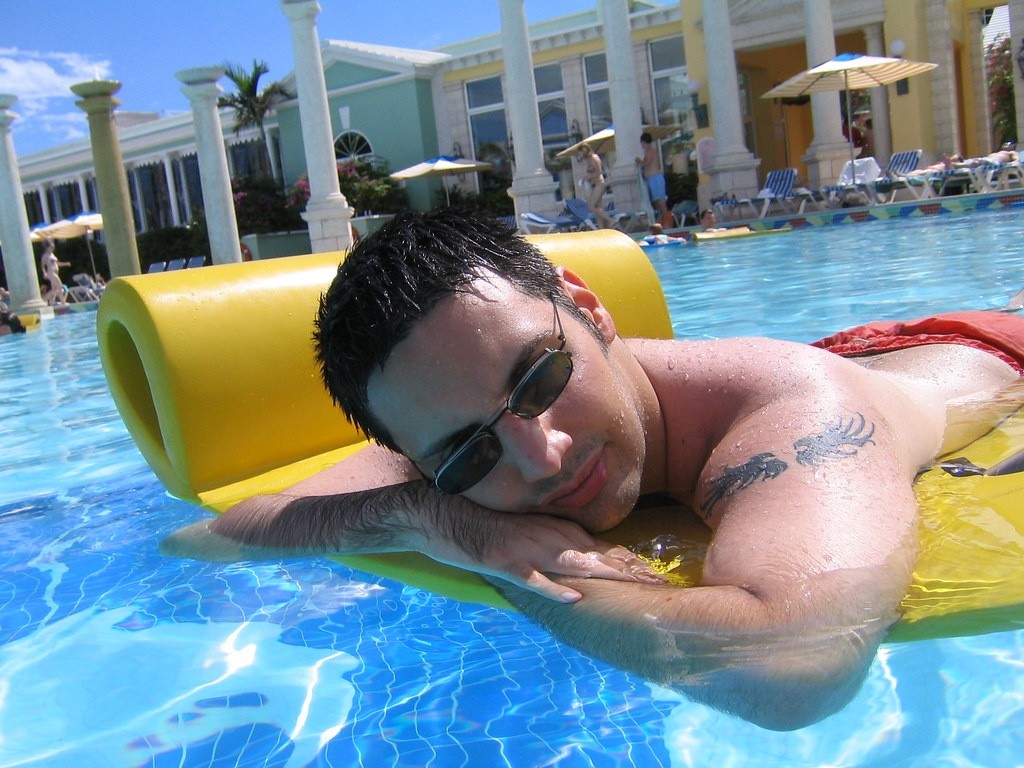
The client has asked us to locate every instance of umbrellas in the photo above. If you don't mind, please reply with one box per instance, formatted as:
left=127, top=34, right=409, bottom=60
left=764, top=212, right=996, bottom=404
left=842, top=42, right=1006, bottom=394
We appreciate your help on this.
left=30, top=211, right=104, bottom=288
left=759, top=53, right=940, bottom=184
left=390, top=156, right=492, bottom=206
left=554, top=121, right=683, bottom=157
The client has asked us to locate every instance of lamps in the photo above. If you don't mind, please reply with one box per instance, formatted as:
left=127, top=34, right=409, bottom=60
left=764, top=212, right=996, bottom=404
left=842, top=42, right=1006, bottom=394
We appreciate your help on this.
left=889, top=39, right=909, bottom=96
left=687, top=79, right=711, bottom=130
left=566, top=118, right=584, bottom=151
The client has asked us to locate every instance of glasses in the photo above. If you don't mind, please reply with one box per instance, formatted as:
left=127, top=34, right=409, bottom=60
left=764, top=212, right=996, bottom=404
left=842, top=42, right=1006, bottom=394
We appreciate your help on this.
left=409, top=291, right=573, bottom=494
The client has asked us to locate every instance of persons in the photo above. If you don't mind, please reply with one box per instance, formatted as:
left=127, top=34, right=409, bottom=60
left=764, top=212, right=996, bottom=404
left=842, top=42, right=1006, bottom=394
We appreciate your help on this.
left=699, top=209, right=727, bottom=233
left=207, top=203, right=1023, bottom=587
left=41, top=241, right=70, bottom=304
left=636, top=224, right=686, bottom=244
left=0, top=288, right=26, bottom=333
left=886, top=137, right=1020, bottom=180
left=636, top=132, right=672, bottom=229
left=570, top=143, right=618, bottom=230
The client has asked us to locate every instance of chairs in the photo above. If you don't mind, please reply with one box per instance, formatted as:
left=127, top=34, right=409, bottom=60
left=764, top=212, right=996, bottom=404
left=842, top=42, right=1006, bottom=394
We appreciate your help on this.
left=495, top=149, right=1024, bottom=234
left=69, top=256, right=206, bottom=302
